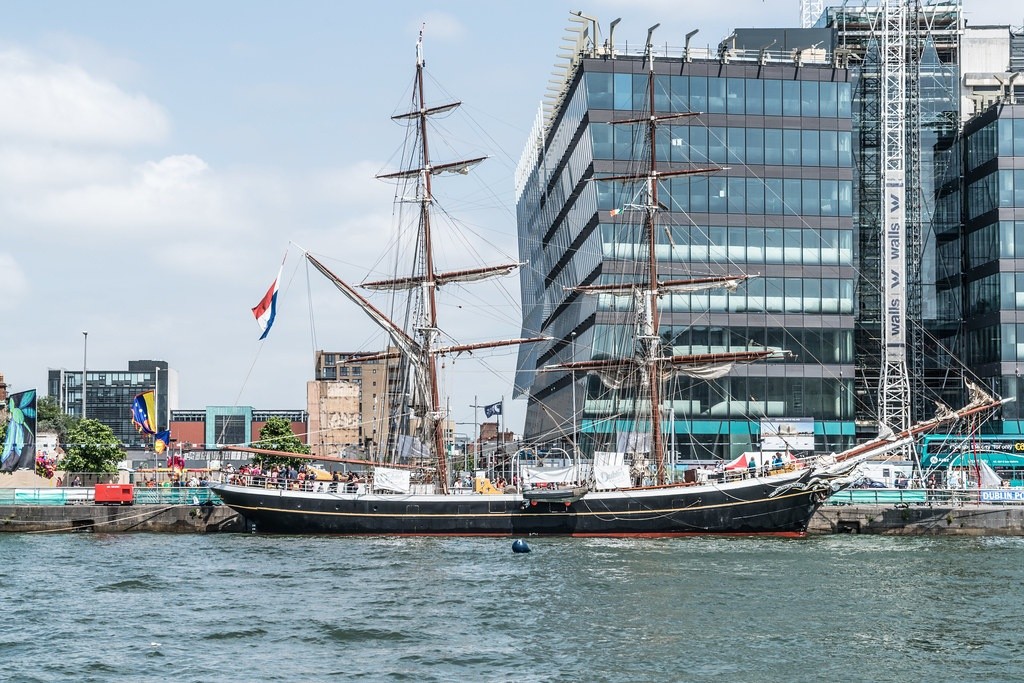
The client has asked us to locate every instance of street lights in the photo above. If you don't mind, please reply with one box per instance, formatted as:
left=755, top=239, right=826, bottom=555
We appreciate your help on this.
left=81, top=330, right=89, bottom=420
left=176, top=441, right=182, bottom=492
left=152, top=453, right=159, bottom=500
left=570, top=331, right=577, bottom=465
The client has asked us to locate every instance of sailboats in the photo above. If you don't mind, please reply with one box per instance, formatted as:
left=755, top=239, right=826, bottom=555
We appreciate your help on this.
left=200, top=20, right=1020, bottom=538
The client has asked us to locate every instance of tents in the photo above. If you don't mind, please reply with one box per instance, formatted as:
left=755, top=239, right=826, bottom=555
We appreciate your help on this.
left=725, top=451, right=799, bottom=473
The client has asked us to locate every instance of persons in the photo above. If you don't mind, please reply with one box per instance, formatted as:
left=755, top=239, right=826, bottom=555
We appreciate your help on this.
left=772, top=452, right=784, bottom=469
left=145, top=477, right=154, bottom=487
left=225, top=461, right=316, bottom=492
left=71, top=476, right=81, bottom=487
left=698, top=460, right=724, bottom=472
left=453, top=474, right=472, bottom=493
left=749, top=456, right=756, bottom=478
left=186, top=476, right=208, bottom=487
left=57, top=477, right=62, bottom=487
left=333, top=470, right=374, bottom=483
left=926, top=474, right=940, bottom=506
left=764, top=460, right=770, bottom=472
left=895, top=472, right=908, bottom=489
left=174, top=479, right=179, bottom=487
left=493, top=474, right=616, bottom=494
left=317, top=483, right=324, bottom=492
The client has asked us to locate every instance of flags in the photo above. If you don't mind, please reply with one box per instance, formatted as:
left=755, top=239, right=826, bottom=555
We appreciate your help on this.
left=252, top=265, right=279, bottom=341
left=485, top=402, right=502, bottom=418
left=154, top=430, right=170, bottom=454
left=0, top=389, right=36, bottom=472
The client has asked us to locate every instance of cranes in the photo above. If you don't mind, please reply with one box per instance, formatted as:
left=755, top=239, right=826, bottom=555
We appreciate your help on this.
left=875, top=0, right=916, bottom=462
